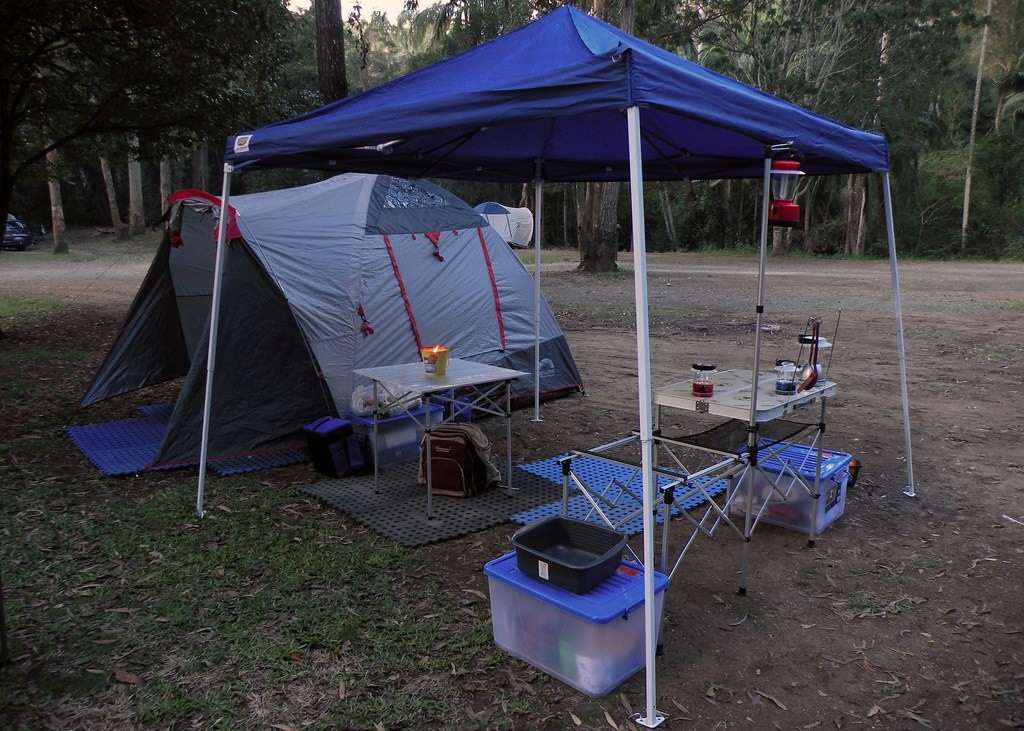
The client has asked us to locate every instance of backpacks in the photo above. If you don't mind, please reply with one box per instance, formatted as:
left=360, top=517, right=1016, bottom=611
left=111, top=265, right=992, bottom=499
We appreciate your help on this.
left=418, top=420, right=501, bottom=497
left=304, top=416, right=366, bottom=478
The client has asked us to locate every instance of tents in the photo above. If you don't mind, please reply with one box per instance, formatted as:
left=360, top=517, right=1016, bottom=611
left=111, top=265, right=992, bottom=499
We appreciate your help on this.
left=63, top=169, right=589, bottom=476
left=194, top=0, right=916, bottom=731
left=472, top=201, right=535, bottom=249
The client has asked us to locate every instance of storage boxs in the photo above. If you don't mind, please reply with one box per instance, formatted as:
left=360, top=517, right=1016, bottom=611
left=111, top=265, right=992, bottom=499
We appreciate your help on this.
left=483, top=550, right=668, bottom=700
left=847, top=458, right=862, bottom=487
left=510, top=516, right=628, bottom=596
left=729, top=438, right=852, bottom=537
left=345, top=402, right=447, bottom=471
left=304, top=416, right=364, bottom=479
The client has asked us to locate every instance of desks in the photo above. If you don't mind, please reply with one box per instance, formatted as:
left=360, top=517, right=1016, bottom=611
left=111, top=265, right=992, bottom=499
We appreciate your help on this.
left=351, top=358, right=530, bottom=522
left=557, top=368, right=838, bottom=657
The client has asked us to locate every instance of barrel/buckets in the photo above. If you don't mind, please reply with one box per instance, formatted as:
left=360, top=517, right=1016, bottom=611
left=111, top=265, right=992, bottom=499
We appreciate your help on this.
left=420, top=346, right=450, bottom=377
left=847, top=458, right=863, bottom=487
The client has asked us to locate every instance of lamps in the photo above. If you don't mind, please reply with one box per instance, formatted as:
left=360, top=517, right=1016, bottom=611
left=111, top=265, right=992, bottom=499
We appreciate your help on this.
left=768, top=149, right=805, bottom=227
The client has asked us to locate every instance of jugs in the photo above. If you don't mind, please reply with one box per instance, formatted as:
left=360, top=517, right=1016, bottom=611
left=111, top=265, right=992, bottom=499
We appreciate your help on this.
left=797, top=362, right=825, bottom=387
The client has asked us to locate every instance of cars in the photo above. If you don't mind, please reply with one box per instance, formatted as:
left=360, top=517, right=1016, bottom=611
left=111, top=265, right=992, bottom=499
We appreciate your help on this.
left=3, top=220, right=33, bottom=252
